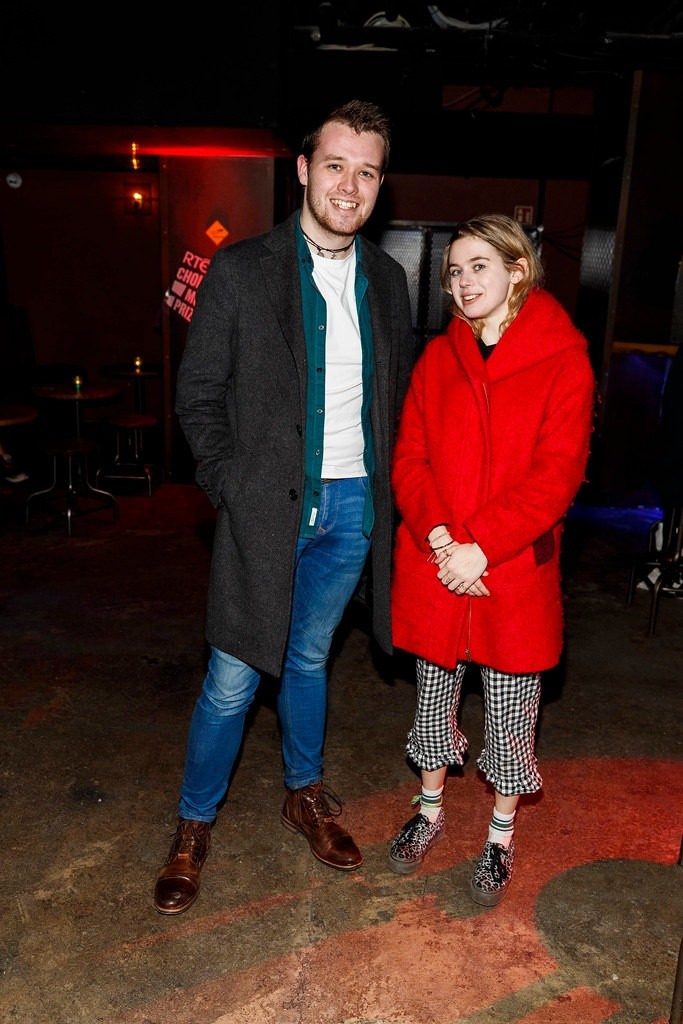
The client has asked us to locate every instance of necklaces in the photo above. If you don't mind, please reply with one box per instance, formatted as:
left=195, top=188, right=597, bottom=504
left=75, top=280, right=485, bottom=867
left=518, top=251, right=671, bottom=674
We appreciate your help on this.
left=300, top=224, right=356, bottom=260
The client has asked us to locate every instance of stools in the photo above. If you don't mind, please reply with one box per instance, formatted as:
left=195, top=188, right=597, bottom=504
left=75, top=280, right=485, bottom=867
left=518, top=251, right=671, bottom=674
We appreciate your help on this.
left=104, top=415, right=163, bottom=497
left=24, top=439, right=120, bottom=538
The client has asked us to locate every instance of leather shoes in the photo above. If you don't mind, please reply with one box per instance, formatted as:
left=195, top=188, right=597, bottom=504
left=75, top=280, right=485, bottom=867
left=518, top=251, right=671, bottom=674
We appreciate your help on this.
left=280, top=782, right=363, bottom=870
left=154, top=815, right=209, bottom=914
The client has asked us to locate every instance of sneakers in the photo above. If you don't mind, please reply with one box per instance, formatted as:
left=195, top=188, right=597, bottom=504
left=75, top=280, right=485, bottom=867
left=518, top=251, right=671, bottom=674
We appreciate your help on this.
left=387, top=807, right=445, bottom=873
left=470, top=838, right=515, bottom=906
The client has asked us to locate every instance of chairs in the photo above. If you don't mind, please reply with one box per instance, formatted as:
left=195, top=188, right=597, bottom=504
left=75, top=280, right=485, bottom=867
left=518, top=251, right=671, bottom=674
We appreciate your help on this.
left=564, top=341, right=683, bottom=639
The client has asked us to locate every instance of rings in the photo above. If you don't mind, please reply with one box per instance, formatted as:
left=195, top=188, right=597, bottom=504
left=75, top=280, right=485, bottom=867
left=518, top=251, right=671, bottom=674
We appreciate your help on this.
left=445, top=575, right=453, bottom=583
left=443, top=550, right=449, bottom=556
left=460, top=585, right=466, bottom=590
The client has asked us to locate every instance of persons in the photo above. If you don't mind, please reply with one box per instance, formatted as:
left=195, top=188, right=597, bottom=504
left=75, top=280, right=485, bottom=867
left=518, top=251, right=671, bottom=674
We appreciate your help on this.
left=155, top=98, right=419, bottom=912
left=389, top=214, right=592, bottom=906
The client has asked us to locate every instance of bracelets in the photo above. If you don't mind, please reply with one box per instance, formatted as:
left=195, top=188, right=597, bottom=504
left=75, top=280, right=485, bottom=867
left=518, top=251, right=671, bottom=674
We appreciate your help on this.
left=429, top=533, right=455, bottom=550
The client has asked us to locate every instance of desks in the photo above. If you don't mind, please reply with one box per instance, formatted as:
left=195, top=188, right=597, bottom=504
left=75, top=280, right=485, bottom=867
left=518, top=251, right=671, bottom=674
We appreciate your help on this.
left=0, top=361, right=165, bottom=479
